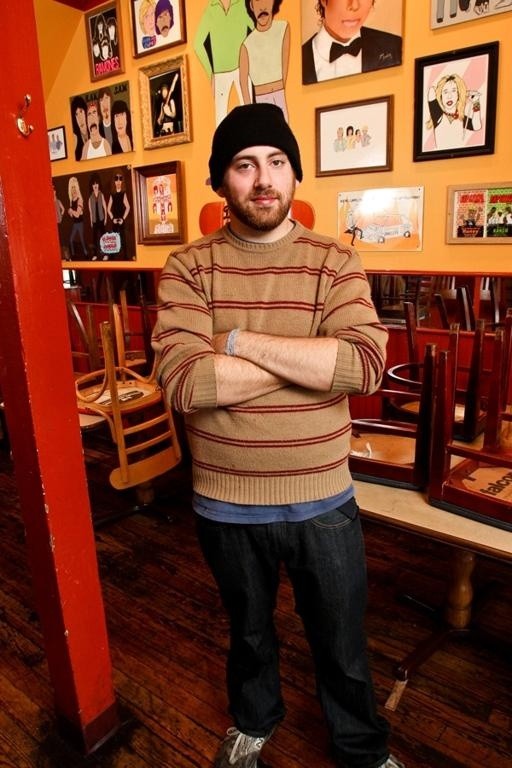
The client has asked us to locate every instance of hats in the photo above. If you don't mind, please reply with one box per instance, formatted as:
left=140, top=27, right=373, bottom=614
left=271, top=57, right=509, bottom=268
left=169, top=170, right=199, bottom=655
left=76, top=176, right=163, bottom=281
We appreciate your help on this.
left=209, top=102, right=302, bottom=190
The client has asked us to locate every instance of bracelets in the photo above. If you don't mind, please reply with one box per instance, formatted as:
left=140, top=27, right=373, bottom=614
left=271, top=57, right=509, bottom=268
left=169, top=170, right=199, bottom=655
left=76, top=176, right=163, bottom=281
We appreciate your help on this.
left=122, top=217, right=125, bottom=221
left=224, top=325, right=241, bottom=354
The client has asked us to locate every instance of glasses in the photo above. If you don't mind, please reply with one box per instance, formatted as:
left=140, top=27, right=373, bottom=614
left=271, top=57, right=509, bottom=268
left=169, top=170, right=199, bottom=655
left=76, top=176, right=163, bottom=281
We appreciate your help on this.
left=114, top=176, right=122, bottom=180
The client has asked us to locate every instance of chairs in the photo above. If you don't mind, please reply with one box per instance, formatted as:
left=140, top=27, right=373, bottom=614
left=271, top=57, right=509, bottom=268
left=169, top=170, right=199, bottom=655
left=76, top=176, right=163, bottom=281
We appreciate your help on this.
left=349, top=275, right=512, bottom=533
left=65, top=270, right=181, bottom=490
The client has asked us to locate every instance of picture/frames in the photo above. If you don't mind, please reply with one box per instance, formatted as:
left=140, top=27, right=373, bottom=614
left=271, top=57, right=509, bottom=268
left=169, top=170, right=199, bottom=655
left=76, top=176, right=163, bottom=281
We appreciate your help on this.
left=413, top=40, right=499, bottom=162
left=133, top=160, right=187, bottom=245
left=84, top=0, right=126, bottom=82
left=129, top=0, right=187, bottom=58
left=138, top=54, right=191, bottom=150
left=445, top=181, right=512, bottom=244
left=48, top=125, right=67, bottom=162
left=315, top=94, right=395, bottom=176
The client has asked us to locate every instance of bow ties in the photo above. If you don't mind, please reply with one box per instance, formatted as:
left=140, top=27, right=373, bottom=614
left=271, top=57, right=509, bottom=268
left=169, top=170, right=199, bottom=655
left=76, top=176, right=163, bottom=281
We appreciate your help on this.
left=329, top=36, right=362, bottom=62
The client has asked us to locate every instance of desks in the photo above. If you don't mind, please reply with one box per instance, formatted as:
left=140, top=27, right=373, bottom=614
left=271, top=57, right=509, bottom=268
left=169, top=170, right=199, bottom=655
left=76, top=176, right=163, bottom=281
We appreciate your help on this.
left=353, top=431, right=512, bottom=681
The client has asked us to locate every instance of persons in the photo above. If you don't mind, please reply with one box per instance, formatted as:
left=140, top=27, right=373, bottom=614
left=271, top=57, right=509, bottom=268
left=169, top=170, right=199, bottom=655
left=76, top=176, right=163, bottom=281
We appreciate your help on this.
left=139, top=0, right=156, bottom=33
left=52, top=182, right=65, bottom=262
left=100, top=40, right=112, bottom=61
left=427, top=74, right=482, bottom=150
left=91, top=15, right=106, bottom=41
left=505, top=205, right=512, bottom=224
left=55, top=134, right=62, bottom=149
left=346, top=126, right=355, bottom=148
left=361, top=126, right=372, bottom=145
left=302, top=0, right=402, bottom=84
left=106, top=17, right=118, bottom=44
left=92, top=39, right=100, bottom=57
left=50, top=134, right=56, bottom=152
left=492, top=209, right=503, bottom=223
left=488, top=205, right=496, bottom=226
left=168, top=201, right=172, bottom=212
left=110, top=100, right=132, bottom=154
left=107, top=168, right=130, bottom=259
left=356, top=128, right=361, bottom=142
left=159, top=198, right=165, bottom=210
left=158, top=183, right=164, bottom=195
left=459, top=205, right=483, bottom=225
left=67, top=176, right=88, bottom=258
left=148, top=104, right=406, bottom=761
left=239, top=0, right=291, bottom=126
left=194, top=0, right=256, bottom=128
left=153, top=185, right=157, bottom=193
left=154, top=0, right=174, bottom=37
left=152, top=202, right=157, bottom=213
left=344, top=207, right=364, bottom=246
left=332, top=126, right=346, bottom=153
left=156, top=83, right=177, bottom=135
left=97, top=87, right=114, bottom=147
left=155, top=209, right=170, bottom=225
left=79, top=92, right=112, bottom=159
left=70, top=95, right=90, bottom=161
left=86, top=173, right=109, bottom=260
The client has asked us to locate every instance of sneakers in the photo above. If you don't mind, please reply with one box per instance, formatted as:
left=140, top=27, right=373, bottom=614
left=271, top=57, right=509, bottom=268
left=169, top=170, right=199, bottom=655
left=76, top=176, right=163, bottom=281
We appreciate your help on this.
left=213, top=726, right=275, bottom=767
